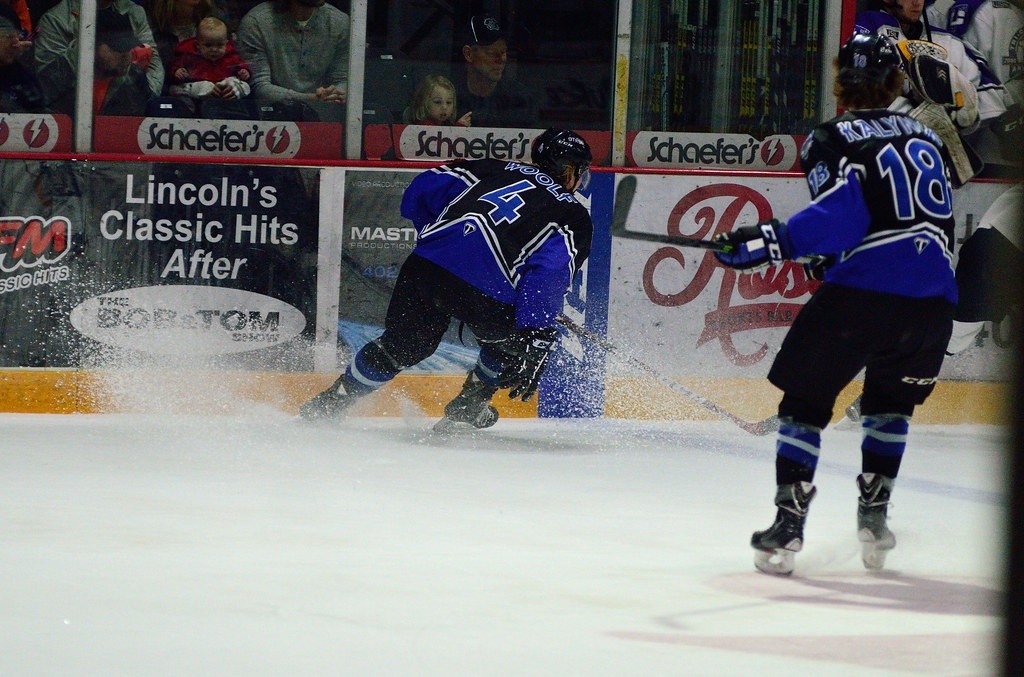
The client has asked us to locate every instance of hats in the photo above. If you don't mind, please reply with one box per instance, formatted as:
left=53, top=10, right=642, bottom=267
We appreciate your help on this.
left=462, top=15, right=511, bottom=46
left=96, top=8, right=145, bottom=53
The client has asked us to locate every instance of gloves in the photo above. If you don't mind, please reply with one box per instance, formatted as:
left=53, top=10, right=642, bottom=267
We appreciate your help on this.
left=804, top=254, right=836, bottom=280
left=713, top=219, right=790, bottom=269
left=498, top=327, right=561, bottom=401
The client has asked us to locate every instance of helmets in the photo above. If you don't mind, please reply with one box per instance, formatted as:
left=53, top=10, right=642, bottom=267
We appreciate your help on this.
left=531, top=129, right=591, bottom=190
left=838, top=35, right=904, bottom=84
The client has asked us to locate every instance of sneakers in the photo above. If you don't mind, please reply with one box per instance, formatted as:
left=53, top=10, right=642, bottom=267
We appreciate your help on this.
left=856, top=473, right=896, bottom=570
left=751, top=481, right=816, bottom=576
left=432, top=371, right=499, bottom=432
left=300, top=374, right=368, bottom=424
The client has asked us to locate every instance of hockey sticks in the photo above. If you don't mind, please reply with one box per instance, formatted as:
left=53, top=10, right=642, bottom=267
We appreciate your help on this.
left=559, top=312, right=781, bottom=438
left=607, top=175, right=827, bottom=267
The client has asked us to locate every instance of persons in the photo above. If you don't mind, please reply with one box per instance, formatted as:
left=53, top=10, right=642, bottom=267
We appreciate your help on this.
left=399, top=72, right=473, bottom=126
left=451, top=11, right=534, bottom=128
left=297, top=127, right=594, bottom=429
left=234, top=0, right=352, bottom=120
left=1, top=0, right=165, bottom=114
left=169, top=15, right=252, bottom=118
left=709, top=32, right=959, bottom=578
left=834, top=178, right=1024, bottom=443
left=854, top=0, right=1024, bottom=189
